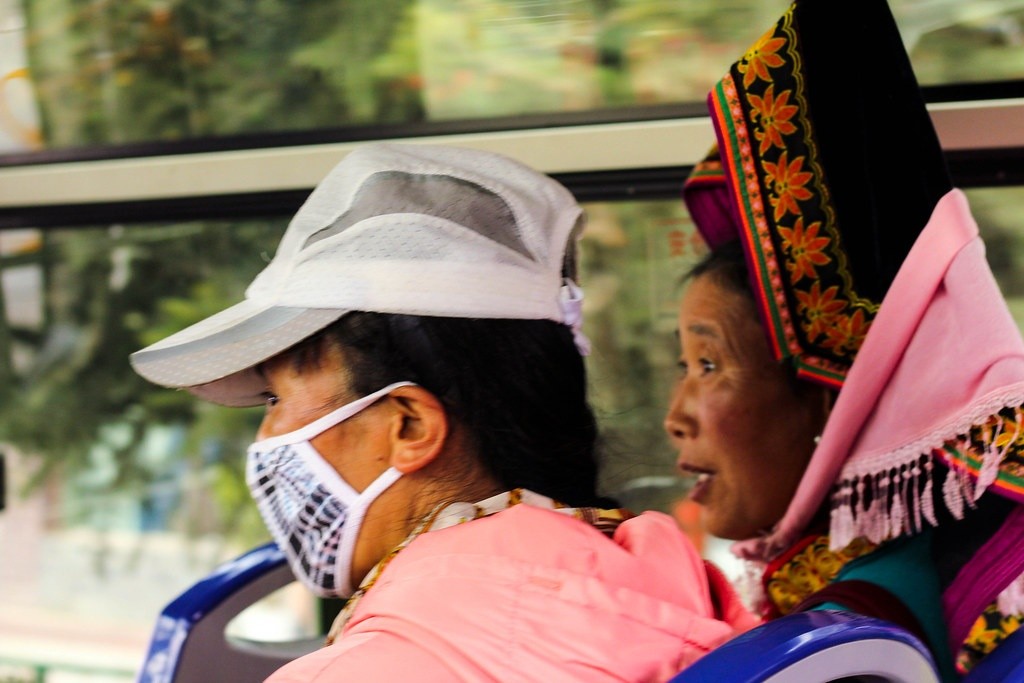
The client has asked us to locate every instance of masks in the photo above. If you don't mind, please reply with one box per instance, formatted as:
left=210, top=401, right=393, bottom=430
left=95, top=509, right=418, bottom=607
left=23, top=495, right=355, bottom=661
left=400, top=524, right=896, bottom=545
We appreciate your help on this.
left=244, top=380, right=415, bottom=598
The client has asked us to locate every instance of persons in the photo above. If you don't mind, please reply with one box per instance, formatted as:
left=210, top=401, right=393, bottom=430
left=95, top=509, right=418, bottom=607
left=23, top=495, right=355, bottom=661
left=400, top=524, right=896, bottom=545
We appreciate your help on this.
left=129, top=141, right=760, bottom=683
left=666, top=1, right=1024, bottom=683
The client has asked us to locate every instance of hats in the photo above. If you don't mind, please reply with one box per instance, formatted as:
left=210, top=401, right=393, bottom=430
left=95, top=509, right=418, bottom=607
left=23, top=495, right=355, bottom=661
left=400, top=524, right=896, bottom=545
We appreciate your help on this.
left=127, top=142, right=588, bottom=407
left=684, top=0, right=1024, bottom=506
